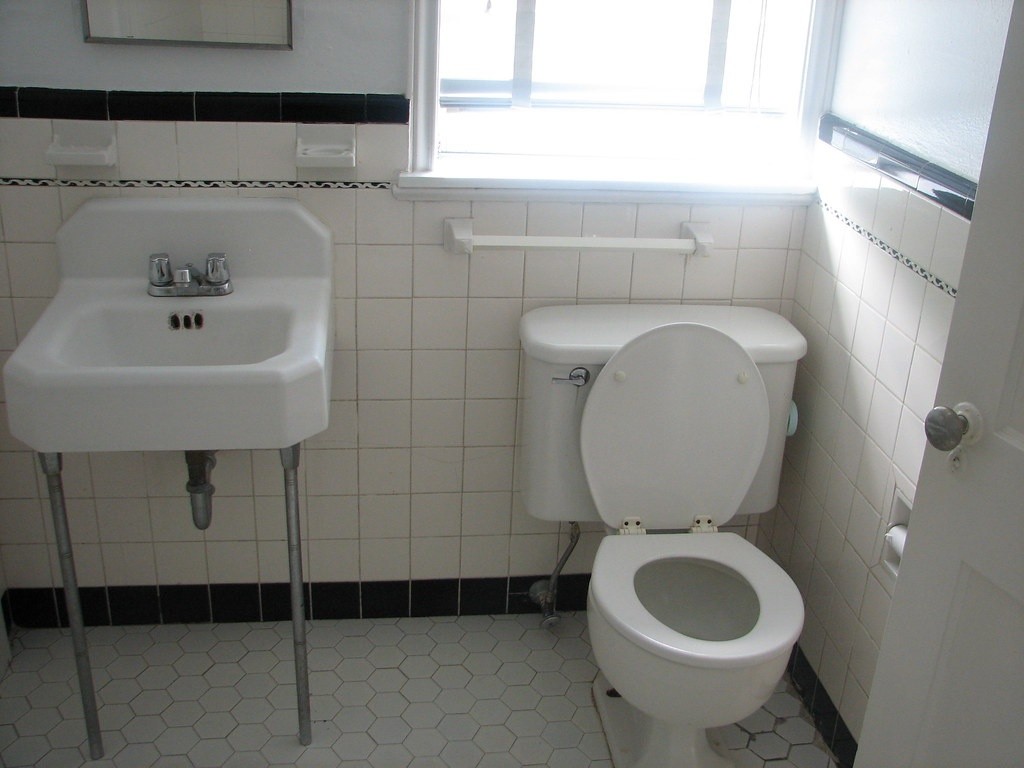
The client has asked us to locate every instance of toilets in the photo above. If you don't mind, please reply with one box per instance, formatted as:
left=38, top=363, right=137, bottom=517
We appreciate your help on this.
left=515, top=301, right=811, bottom=768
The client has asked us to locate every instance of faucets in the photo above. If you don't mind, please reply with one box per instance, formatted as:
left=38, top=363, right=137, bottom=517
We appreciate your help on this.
left=148, top=250, right=234, bottom=297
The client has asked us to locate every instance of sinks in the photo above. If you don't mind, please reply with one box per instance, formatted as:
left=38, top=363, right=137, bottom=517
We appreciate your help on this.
left=3, top=278, right=338, bottom=454
